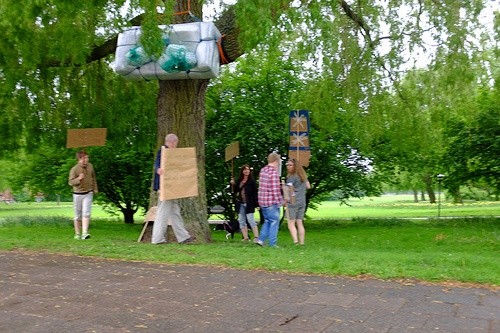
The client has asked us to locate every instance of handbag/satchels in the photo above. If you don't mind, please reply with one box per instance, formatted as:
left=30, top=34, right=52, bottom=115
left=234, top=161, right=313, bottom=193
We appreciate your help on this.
left=235, top=199, right=241, bottom=212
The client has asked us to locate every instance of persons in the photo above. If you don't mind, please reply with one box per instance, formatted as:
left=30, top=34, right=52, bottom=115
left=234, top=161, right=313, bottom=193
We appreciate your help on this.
left=69, top=152, right=98, bottom=239
left=256, top=153, right=285, bottom=248
left=230, top=164, right=259, bottom=242
left=283, top=158, right=311, bottom=246
left=151, top=134, right=197, bottom=245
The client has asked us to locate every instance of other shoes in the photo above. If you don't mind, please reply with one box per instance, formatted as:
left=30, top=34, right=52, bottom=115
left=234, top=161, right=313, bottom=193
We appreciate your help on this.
left=257, top=240, right=265, bottom=246
left=74, top=234, right=79, bottom=240
left=253, top=238, right=258, bottom=243
left=181, top=237, right=197, bottom=244
left=241, top=238, right=250, bottom=241
left=82, top=233, right=91, bottom=239
